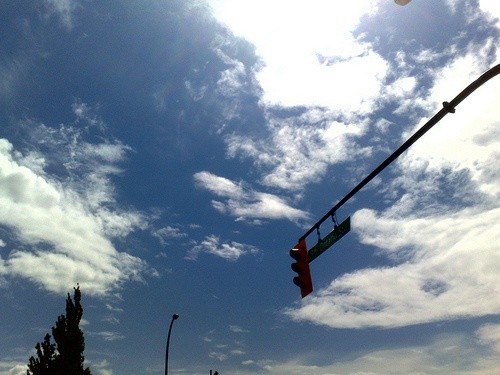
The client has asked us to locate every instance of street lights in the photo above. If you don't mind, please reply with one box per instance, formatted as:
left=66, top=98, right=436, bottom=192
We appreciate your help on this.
left=164, top=313, right=180, bottom=375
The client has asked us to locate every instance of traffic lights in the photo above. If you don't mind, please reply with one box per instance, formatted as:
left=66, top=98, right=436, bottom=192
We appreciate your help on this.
left=289, top=235, right=312, bottom=296
